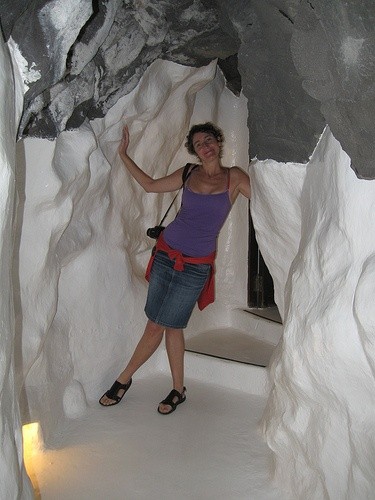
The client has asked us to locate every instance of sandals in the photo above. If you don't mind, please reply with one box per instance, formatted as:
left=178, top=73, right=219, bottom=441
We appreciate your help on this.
left=99, top=378, right=132, bottom=406
left=157, top=386, right=186, bottom=414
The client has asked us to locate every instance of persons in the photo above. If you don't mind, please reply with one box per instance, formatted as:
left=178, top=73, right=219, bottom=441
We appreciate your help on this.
left=99, top=120, right=255, bottom=415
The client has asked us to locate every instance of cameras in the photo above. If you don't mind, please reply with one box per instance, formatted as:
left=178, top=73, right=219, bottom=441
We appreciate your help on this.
left=146, top=226, right=166, bottom=241
